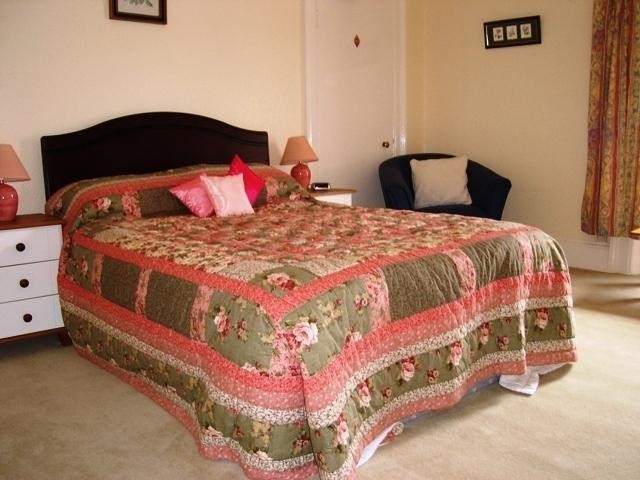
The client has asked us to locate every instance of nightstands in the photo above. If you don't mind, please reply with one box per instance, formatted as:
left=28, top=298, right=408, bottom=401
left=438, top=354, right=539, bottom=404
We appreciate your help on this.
left=0, top=213, right=72, bottom=346
left=307, top=188, right=357, bottom=207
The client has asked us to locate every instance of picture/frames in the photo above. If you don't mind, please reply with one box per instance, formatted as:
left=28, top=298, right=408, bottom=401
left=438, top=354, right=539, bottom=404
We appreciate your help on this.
left=109, top=0, right=167, bottom=25
left=483, top=15, right=541, bottom=49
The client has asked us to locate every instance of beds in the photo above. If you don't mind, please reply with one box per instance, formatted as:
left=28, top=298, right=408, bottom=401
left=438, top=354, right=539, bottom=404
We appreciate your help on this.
left=40, top=111, right=578, bottom=480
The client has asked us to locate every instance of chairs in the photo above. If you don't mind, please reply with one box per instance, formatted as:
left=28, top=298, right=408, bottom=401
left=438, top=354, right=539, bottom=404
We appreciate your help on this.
left=378, top=152, right=512, bottom=220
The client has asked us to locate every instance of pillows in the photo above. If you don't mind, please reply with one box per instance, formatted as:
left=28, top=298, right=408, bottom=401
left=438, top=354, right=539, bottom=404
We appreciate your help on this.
left=169, top=153, right=267, bottom=218
left=410, top=155, right=472, bottom=209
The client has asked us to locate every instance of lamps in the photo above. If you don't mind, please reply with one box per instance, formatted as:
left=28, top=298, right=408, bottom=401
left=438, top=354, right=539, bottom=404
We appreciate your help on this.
left=279, top=136, right=319, bottom=191
left=0, top=144, right=31, bottom=222
